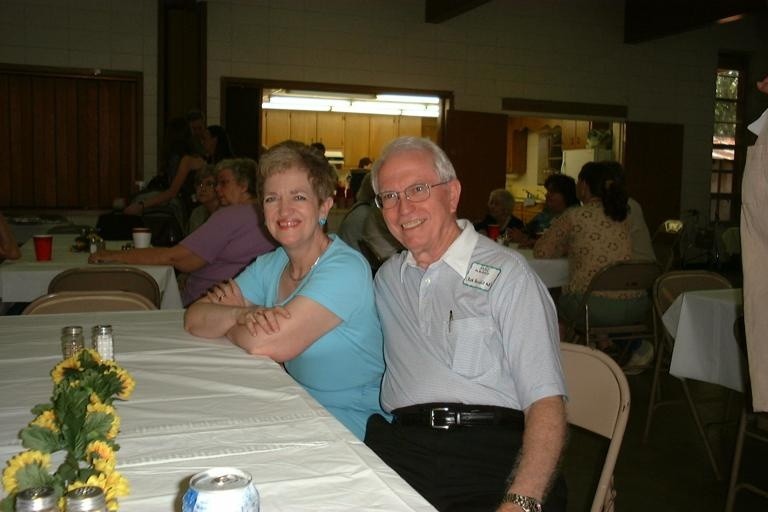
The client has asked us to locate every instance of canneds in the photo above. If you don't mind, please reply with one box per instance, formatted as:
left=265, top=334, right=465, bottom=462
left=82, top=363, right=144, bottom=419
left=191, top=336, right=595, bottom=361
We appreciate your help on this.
left=181, top=466, right=260, bottom=512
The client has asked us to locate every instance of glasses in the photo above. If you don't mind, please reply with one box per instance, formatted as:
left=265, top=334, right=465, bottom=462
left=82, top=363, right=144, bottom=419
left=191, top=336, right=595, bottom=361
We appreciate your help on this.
left=375, top=183, right=449, bottom=209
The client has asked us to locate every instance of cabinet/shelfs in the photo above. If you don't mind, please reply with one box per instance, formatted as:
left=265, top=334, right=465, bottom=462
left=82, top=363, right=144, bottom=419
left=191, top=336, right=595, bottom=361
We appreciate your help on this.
left=522, top=200, right=545, bottom=228
left=536, top=127, right=564, bottom=186
left=560, top=119, right=609, bottom=151
left=261, top=107, right=441, bottom=172
left=506, top=128, right=530, bottom=175
left=512, top=199, right=522, bottom=219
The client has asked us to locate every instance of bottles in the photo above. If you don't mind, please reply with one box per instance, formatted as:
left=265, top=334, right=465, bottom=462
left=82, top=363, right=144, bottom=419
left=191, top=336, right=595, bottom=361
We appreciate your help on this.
left=92, top=324, right=114, bottom=363
left=88, top=237, right=105, bottom=262
left=14, top=487, right=61, bottom=512
left=63, top=486, right=107, bottom=512
left=134, top=180, right=146, bottom=195
left=60, top=326, right=85, bottom=357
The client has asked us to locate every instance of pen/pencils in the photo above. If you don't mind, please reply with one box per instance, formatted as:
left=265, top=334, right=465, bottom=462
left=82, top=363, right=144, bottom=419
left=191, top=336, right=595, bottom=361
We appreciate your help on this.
left=449, top=310, right=453, bottom=333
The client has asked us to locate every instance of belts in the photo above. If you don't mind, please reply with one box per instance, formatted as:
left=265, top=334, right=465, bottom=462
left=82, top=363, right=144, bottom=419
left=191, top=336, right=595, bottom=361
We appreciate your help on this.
left=393, top=407, right=523, bottom=430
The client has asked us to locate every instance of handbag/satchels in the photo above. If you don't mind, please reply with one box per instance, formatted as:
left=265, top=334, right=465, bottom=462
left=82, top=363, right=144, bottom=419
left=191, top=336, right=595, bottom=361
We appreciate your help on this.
left=97, top=212, right=144, bottom=241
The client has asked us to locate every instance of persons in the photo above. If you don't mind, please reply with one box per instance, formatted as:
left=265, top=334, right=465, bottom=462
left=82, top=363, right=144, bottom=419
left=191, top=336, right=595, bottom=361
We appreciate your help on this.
left=370, top=136, right=569, bottom=512
left=0, top=213, right=22, bottom=259
left=184, top=140, right=393, bottom=442
left=739, top=76, right=767, bottom=415
left=89, top=123, right=657, bottom=365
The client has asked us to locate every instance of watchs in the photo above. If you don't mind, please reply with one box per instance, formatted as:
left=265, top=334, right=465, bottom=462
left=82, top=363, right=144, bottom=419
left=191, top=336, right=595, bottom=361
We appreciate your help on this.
left=502, top=493, right=542, bottom=512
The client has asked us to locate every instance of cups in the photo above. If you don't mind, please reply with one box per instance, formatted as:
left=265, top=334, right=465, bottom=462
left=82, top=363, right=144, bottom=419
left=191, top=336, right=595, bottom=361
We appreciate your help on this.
left=32, top=234, right=52, bottom=261
left=132, top=227, right=151, bottom=233
left=536, top=232, right=544, bottom=239
left=132, top=232, right=152, bottom=249
left=488, top=224, right=500, bottom=242
left=496, top=228, right=513, bottom=247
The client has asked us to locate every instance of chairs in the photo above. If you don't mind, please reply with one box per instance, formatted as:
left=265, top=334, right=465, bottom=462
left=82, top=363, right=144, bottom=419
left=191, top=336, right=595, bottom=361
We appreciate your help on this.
left=20, top=291, right=159, bottom=317
left=515, top=336, right=634, bottom=512
left=43, top=266, right=158, bottom=302
left=563, top=256, right=661, bottom=400
left=643, top=267, right=735, bottom=482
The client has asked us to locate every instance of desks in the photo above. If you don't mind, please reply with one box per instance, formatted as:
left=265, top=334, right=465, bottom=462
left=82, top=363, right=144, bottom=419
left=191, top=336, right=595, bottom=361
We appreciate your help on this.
left=3, top=310, right=768, bottom=512
left=474, top=228, right=572, bottom=295
left=3, top=219, right=185, bottom=304
